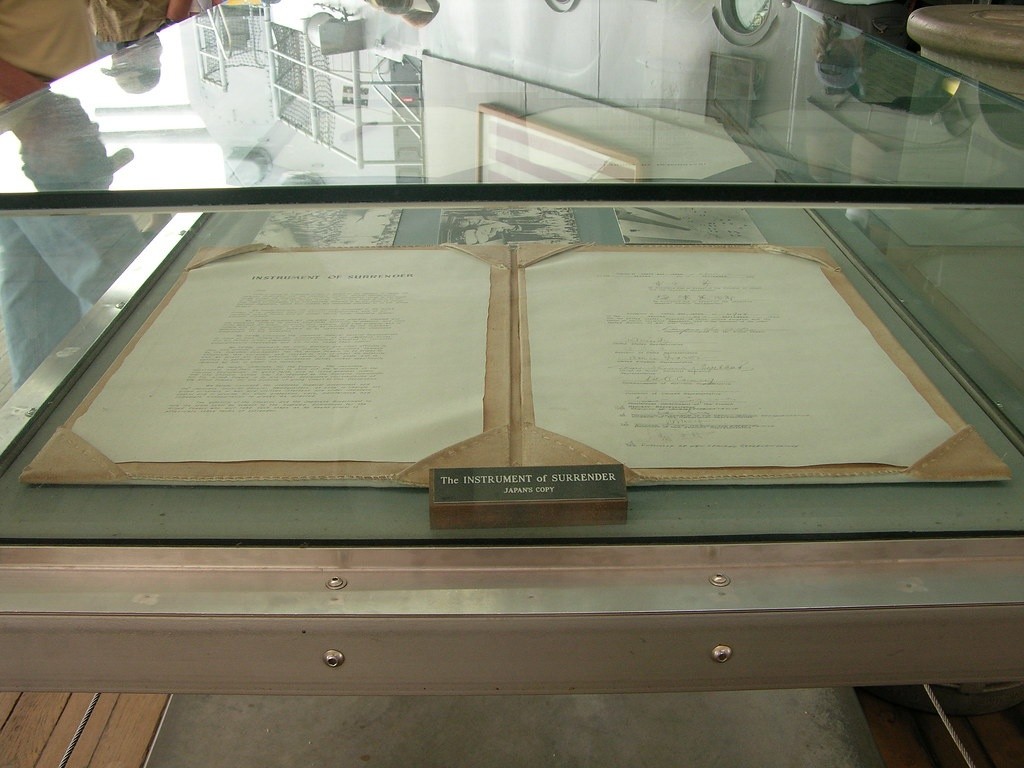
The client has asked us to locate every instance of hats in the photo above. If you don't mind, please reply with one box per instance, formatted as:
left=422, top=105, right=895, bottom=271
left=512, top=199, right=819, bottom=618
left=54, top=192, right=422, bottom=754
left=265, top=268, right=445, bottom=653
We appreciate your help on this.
left=22, top=148, right=135, bottom=190
left=98, top=66, right=161, bottom=95
left=814, top=61, right=862, bottom=89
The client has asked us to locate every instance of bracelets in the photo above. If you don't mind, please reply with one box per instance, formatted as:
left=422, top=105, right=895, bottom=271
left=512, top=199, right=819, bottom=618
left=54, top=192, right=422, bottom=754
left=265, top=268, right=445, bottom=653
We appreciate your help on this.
left=166, top=19, right=173, bottom=25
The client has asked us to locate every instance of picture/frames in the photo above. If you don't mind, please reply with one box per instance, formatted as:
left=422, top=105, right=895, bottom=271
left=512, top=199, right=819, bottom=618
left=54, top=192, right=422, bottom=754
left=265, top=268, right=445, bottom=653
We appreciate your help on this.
left=704, top=52, right=756, bottom=135
left=476, top=103, right=641, bottom=184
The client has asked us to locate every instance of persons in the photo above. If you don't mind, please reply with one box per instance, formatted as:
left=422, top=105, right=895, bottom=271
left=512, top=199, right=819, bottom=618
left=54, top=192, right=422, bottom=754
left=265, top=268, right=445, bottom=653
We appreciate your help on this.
left=101, top=34, right=162, bottom=94
left=0, top=0, right=148, bottom=392
left=0, top=91, right=134, bottom=192
left=86, top=0, right=193, bottom=52
